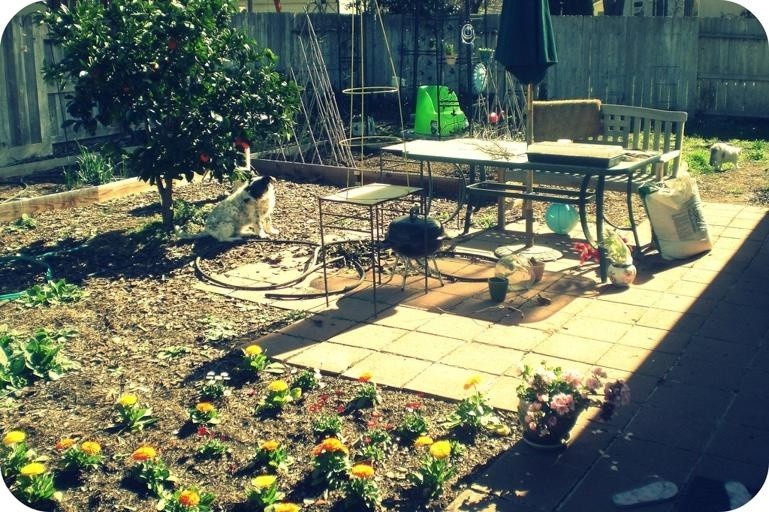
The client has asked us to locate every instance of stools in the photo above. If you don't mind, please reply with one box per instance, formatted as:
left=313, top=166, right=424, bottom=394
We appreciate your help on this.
left=317, top=179, right=425, bottom=319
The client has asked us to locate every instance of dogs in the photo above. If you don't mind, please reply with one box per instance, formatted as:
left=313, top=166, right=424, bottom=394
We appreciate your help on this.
left=173, top=175, right=280, bottom=242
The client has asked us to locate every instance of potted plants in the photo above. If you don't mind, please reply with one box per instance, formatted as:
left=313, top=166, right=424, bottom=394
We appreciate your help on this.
left=594, top=230, right=638, bottom=291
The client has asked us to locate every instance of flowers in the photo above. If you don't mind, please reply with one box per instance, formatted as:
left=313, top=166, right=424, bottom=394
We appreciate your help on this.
left=515, top=357, right=631, bottom=440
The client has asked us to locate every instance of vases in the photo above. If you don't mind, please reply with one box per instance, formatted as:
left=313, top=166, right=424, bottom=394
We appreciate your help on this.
left=522, top=431, right=563, bottom=472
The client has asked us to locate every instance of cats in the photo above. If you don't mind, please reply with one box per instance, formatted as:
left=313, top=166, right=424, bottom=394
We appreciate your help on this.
left=709, top=143, right=743, bottom=172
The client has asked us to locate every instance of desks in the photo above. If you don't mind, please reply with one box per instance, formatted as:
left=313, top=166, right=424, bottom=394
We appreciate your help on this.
left=381, top=138, right=663, bottom=287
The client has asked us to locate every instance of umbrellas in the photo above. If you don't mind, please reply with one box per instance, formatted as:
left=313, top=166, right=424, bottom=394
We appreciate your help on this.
left=492, top=0, right=561, bottom=248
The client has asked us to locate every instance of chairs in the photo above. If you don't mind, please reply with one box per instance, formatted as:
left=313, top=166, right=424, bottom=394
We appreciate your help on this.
left=413, top=82, right=469, bottom=138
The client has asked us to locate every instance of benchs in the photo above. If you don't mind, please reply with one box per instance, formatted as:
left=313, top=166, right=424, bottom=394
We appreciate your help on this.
left=496, top=103, right=689, bottom=245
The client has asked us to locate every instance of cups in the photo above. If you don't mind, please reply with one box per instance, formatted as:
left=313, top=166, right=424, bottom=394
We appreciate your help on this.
left=532, top=262, right=544, bottom=281
left=488, top=277, right=509, bottom=302
